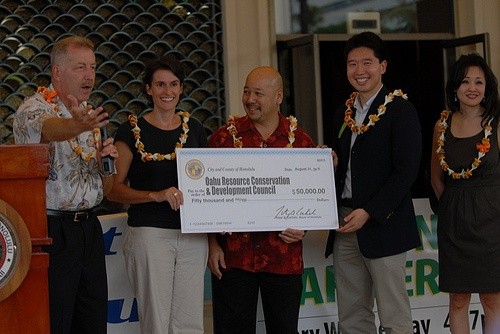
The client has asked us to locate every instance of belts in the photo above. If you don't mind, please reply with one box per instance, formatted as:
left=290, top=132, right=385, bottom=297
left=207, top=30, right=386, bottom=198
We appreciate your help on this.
left=46, top=210, right=95, bottom=222
left=339, top=199, right=352, bottom=206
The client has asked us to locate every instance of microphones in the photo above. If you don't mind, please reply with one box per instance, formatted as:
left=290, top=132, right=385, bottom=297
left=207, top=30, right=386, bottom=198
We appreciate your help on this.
left=100, top=127, right=114, bottom=174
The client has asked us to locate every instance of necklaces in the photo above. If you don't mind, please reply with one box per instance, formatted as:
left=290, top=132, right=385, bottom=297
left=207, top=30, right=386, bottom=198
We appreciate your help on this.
left=127, top=110, right=190, bottom=162
left=436, top=109, right=493, bottom=180
left=226, top=113, right=299, bottom=149
left=344, top=89, right=407, bottom=136
left=36, top=83, right=102, bottom=160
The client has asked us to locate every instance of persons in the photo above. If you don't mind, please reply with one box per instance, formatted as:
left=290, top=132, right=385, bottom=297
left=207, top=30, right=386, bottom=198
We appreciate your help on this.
left=424, top=53, right=500, bottom=334
left=13, top=36, right=119, bottom=334
left=315, top=31, right=423, bottom=334
left=104, top=62, right=233, bottom=334
left=207, top=66, right=316, bottom=334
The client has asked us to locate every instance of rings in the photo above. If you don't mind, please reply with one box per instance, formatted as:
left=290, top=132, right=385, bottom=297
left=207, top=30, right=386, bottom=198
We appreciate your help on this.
left=174, top=193, right=177, bottom=196
